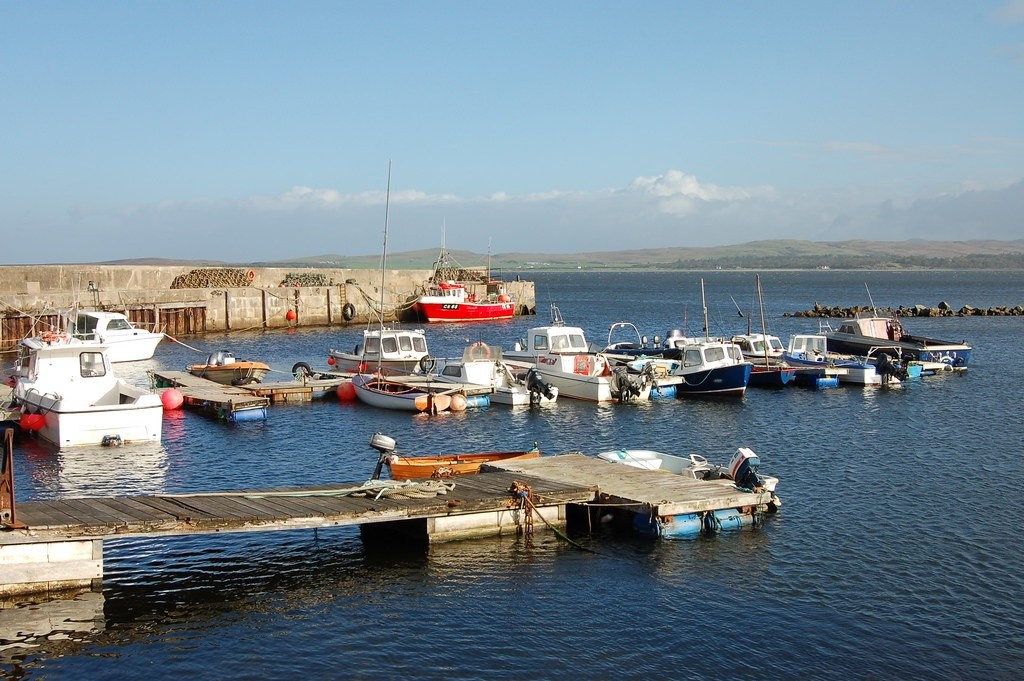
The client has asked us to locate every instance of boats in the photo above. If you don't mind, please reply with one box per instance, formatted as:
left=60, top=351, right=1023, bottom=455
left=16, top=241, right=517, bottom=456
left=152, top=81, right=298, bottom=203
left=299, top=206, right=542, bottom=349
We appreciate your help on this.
left=596, top=347, right=685, bottom=397
left=423, top=331, right=560, bottom=406
left=327, top=326, right=433, bottom=376
left=17, top=280, right=167, bottom=367
left=778, top=333, right=908, bottom=388
left=498, top=298, right=652, bottom=402
left=413, top=217, right=515, bottom=324
left=186, top=348, right=272, bottom=388
left=8, top=324, right=166, bottom=455
left=815, top=281, right=974, bottom=370
left=595, top=446, right=782, bottom=538
left=733, top=331, right=785, bottom=359
left=368, top=433, right=542, bottom=482
left=664, top=276, right=756, bottom=397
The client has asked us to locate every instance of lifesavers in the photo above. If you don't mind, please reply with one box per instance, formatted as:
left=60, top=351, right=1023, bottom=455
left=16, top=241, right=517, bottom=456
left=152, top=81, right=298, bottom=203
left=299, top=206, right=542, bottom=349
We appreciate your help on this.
left=231, top=377, right=249, bottom=385
left=420, top=354, right=437, bottom=373
left=292, top=361, right=311, bottom=379
left=249, top=377, right=261, bottom=384
left=248, top=270, right=255, bottom=281
left=42, top=333, right=66, bottom=341
left=342, top=302, right=356, bottom=321
left=468, top=341, right=490, bottom=361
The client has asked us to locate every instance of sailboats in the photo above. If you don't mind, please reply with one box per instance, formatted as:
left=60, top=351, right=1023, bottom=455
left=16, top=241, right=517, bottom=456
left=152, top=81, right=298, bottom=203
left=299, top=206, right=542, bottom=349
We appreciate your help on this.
left=742, top=272, right=795, bottom=389
left=351, top=157, right=466, bottom=415
